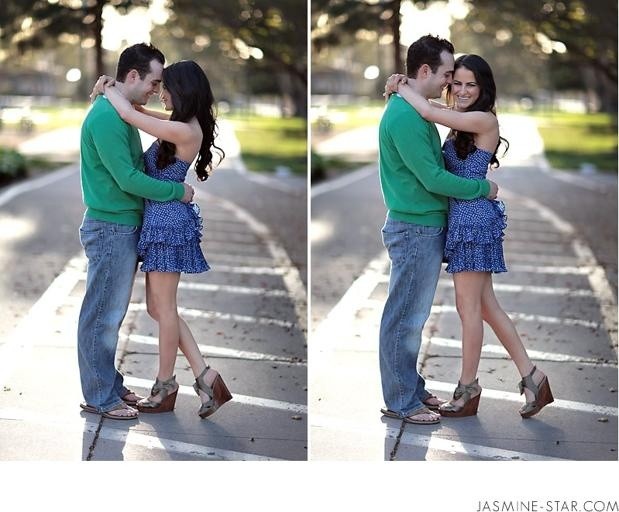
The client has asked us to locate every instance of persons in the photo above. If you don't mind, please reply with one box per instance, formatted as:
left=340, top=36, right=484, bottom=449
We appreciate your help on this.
left=379, top=34, right=555, bottom=425
left=77, top=42, right=233, bottom=420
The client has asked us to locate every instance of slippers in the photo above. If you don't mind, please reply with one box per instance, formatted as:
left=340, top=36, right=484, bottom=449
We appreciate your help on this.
left=80, top=390, right=146, bottom=420
left=381, top=393, right=446, bottom=424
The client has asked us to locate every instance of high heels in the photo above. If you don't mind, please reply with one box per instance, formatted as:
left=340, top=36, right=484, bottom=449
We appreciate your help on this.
left=136, top=375, right=179, bottom=414
left=193, top=365, right=233, bottom=419
left=518, top=365, right=554, bottom=419
left=438, top=378, right=482, bottom=417
